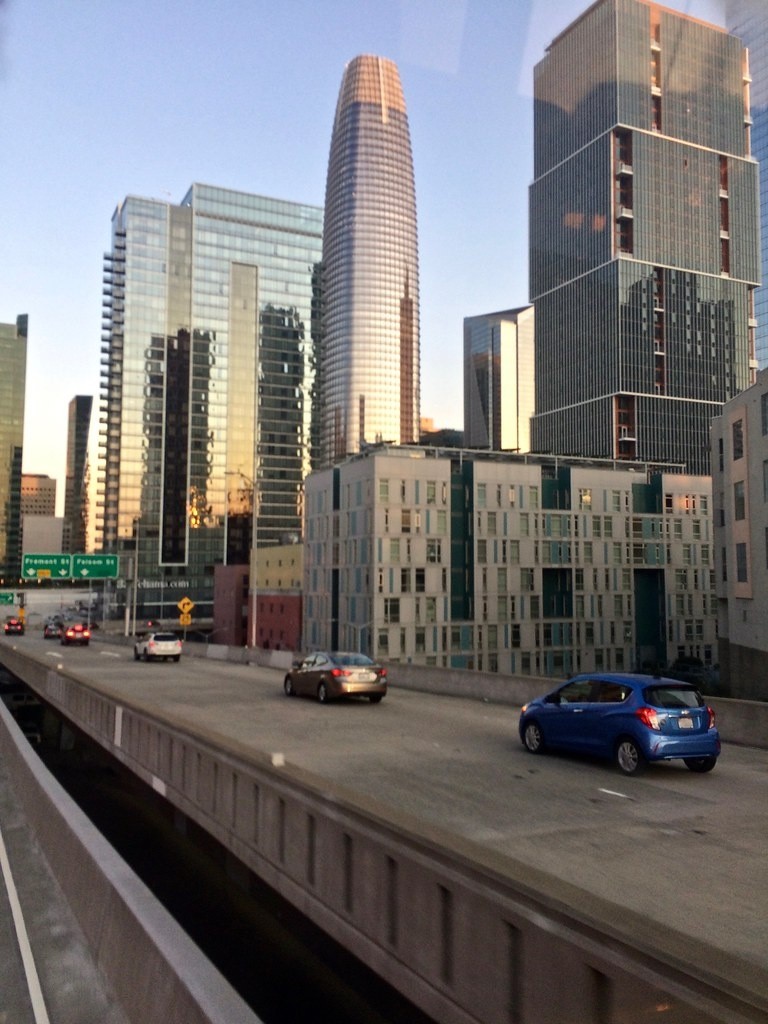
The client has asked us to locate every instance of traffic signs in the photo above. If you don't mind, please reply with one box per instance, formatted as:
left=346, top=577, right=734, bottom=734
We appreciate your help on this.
left=0, top=592, right=13, bottom=605
left=70, top=554, right=119, bottom=580
left=22, top=553, right=72, bottom=580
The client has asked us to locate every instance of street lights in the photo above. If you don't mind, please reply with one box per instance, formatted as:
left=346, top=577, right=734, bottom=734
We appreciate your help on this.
left=121, top=510, right=140, bottom=635
left=224, top=470, right=257, bottom=646
left=191, top=627, right=228, bottom=643
left=345, top=620, right=376, bottom=656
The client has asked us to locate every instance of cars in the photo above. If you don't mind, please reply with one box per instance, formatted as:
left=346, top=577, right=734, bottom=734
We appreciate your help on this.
left=283, top=650, right=387, bottom=703
left=518, top=671, right=722, bottom=774
left=83, top=622, right=100, bottom=630
left=131, top=619, right=161, bottom=636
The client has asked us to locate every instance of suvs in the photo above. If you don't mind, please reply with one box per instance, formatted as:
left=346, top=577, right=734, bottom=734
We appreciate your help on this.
left=5, top=619, right=26, bottom=636
left=61, top=625, right=91, bottom=647
left=44, top=626, right=62, bottom=638
left=133, top=633, right=182, bottom=663
